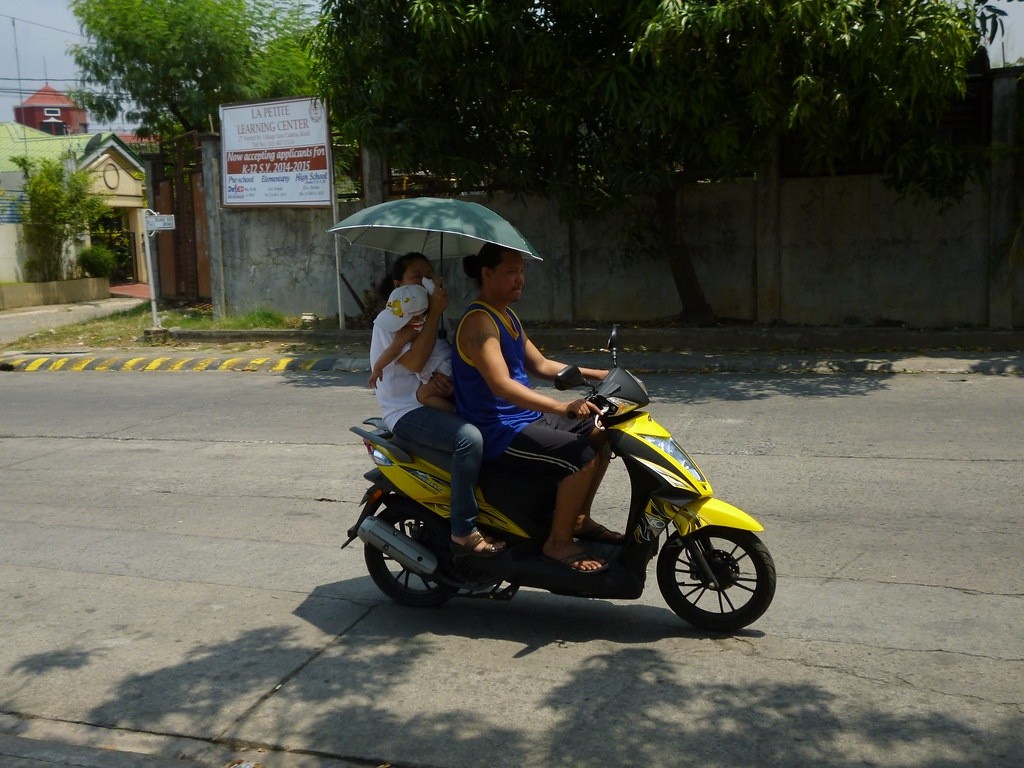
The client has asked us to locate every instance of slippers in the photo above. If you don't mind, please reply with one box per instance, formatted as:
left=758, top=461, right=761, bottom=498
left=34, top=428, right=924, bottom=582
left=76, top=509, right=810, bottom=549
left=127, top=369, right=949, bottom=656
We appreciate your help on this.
left=541, top=552, right=608, bottom=575
left=573, top=525, right=625, bottom=546
left=449, top=530, right=507, bottom=558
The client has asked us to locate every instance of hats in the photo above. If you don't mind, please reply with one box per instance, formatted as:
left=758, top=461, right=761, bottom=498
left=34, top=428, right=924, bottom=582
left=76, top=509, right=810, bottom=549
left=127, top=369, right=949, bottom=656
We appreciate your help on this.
left=373, top=284, right=428, bottom=332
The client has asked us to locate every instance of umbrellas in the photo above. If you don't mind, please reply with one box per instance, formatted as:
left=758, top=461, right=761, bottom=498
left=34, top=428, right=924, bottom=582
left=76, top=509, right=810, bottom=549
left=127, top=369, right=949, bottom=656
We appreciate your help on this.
left=324, top=196, right=544, bottom=328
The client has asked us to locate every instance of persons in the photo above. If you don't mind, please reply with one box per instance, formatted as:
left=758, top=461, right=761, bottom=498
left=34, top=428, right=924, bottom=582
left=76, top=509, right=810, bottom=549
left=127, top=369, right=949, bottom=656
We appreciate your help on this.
left=368, top=285, right=456, bottom=415
left=364, top=252, right=510, bottom=557
left=451, top=243, right=625, bottom=575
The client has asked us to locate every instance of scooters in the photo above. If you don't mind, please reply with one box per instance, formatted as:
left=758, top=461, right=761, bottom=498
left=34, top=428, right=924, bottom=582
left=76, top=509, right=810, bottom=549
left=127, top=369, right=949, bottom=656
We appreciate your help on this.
left=338, top=323, right=779, bottom=632
